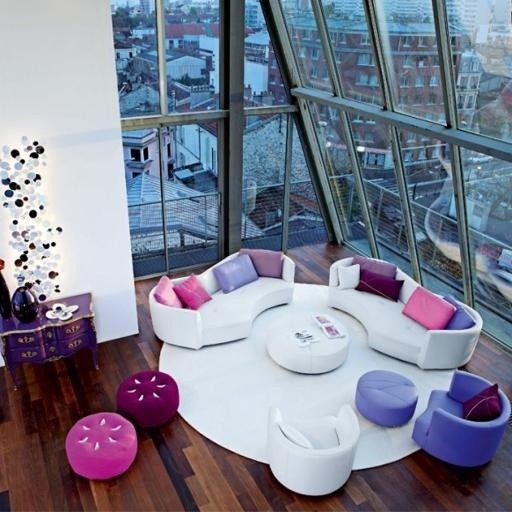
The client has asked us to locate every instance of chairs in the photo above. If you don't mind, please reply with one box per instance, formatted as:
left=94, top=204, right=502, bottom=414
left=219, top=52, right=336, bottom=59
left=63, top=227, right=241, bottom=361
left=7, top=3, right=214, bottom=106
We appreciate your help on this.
left=412, top=370, right=512, bottom=466
left=268, top=404, right=361, bottom=495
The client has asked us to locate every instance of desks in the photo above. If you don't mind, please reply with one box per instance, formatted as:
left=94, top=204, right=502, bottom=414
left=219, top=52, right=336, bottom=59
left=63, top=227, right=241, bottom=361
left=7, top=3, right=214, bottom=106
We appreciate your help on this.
left=266, top=312, right=350, bottom=374
left=0, top=293, right=99, bottom=390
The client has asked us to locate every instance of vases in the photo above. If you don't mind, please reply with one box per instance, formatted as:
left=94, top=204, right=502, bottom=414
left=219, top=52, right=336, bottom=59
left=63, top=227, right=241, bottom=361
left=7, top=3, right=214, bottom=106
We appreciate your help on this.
left=12, top=287, right=39, bottom=323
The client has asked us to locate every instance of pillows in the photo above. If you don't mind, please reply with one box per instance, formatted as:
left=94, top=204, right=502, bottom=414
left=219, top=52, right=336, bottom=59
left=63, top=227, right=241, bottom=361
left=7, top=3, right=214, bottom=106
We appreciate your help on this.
left=337, top=263, right=361, bottom=290
left=351, top=254, right=397, bottom=278
left=153, top=275, right=183, bottom=308
left=277, top=422, right=314, bottom=450
left=402, top=286, right=458, bottom=330
left=442, top=294, right=476, bottom=330
left=212, top=254, right=259, bottom=294
left=355, top=269, right=405, bottom=302
left=240, top=248, right=282, bottom=278
left=171, top=273, right=214, bottom=311
left=462, top=381, right=502, bottom=422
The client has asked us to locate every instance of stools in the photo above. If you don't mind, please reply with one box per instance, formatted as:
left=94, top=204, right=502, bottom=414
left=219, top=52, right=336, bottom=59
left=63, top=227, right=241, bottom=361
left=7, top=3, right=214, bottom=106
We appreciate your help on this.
left=65, top=412, right=139, bottom=481
left=355, top=370, right=418, bottom=428
left=116, top=370, right=180, bottom=429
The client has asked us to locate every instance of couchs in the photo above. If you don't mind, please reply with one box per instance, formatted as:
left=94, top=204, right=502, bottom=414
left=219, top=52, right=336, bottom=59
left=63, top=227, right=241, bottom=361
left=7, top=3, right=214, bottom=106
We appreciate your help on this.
left=327, top=256, right=483, bottom=370
left=147, top=248, right=296, bottom=348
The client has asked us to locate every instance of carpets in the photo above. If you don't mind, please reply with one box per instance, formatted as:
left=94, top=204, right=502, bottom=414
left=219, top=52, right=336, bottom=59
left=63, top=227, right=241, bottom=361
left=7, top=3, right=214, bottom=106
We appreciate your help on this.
left=158, top=283, right=457, bottom=471
